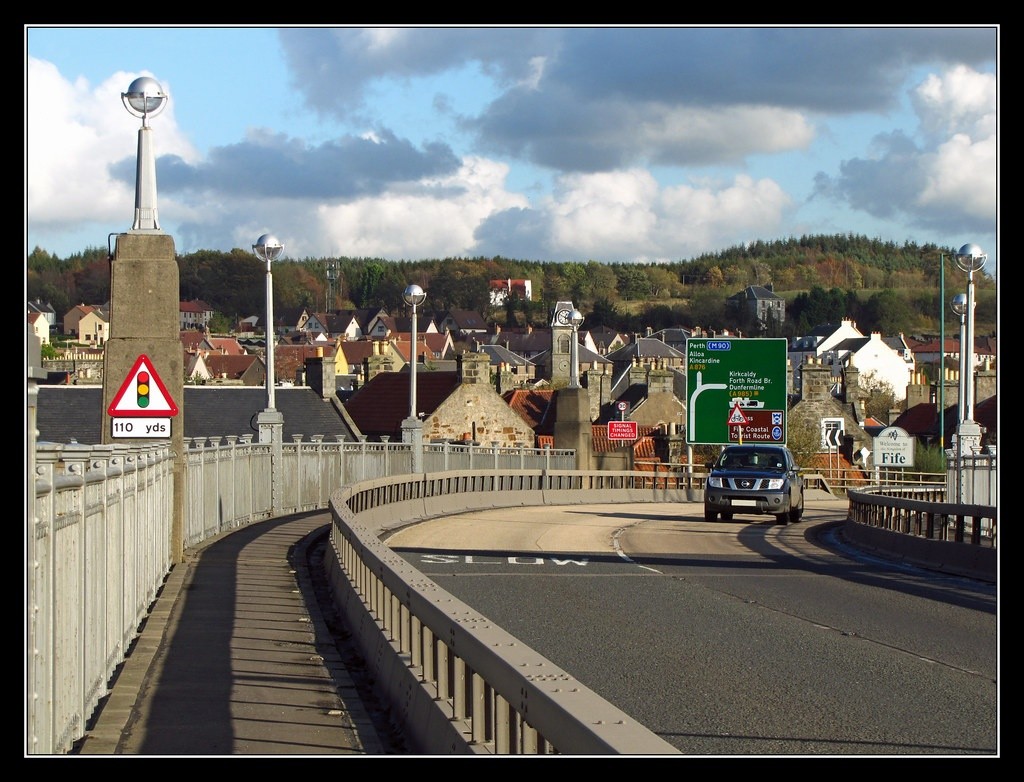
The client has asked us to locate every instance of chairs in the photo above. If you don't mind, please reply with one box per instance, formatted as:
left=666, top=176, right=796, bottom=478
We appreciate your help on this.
left=768, top=456, right=780, bottom=467
left=737, top=455, right=750, bottom=466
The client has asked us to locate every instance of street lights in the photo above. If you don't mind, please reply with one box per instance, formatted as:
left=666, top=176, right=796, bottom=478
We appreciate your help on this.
left=121, top=74, right=170, bottom=233
left=565, top=311, right=584, bottom=388
left=940, top=253, right=958, bottom=473
left=949, top=293, right=978, bottom=423
left=955, top=246, right=989, bottom=422
left=251, top=233, right=284, bottom=408
left=403, top=284, right=426, bottom=417
left=67, top=339, right=70, bottom=349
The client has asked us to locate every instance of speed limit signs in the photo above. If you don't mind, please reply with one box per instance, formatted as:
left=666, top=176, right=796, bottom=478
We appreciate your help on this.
left=619, top=402, right=626, bottom=411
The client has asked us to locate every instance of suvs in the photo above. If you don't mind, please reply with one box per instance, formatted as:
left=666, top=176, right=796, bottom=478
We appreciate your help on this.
left=704, top=446, right=806, bottom=524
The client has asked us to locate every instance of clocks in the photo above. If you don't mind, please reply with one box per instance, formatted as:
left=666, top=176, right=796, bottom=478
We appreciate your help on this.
left=557, top=308, right=573, bottom=325
